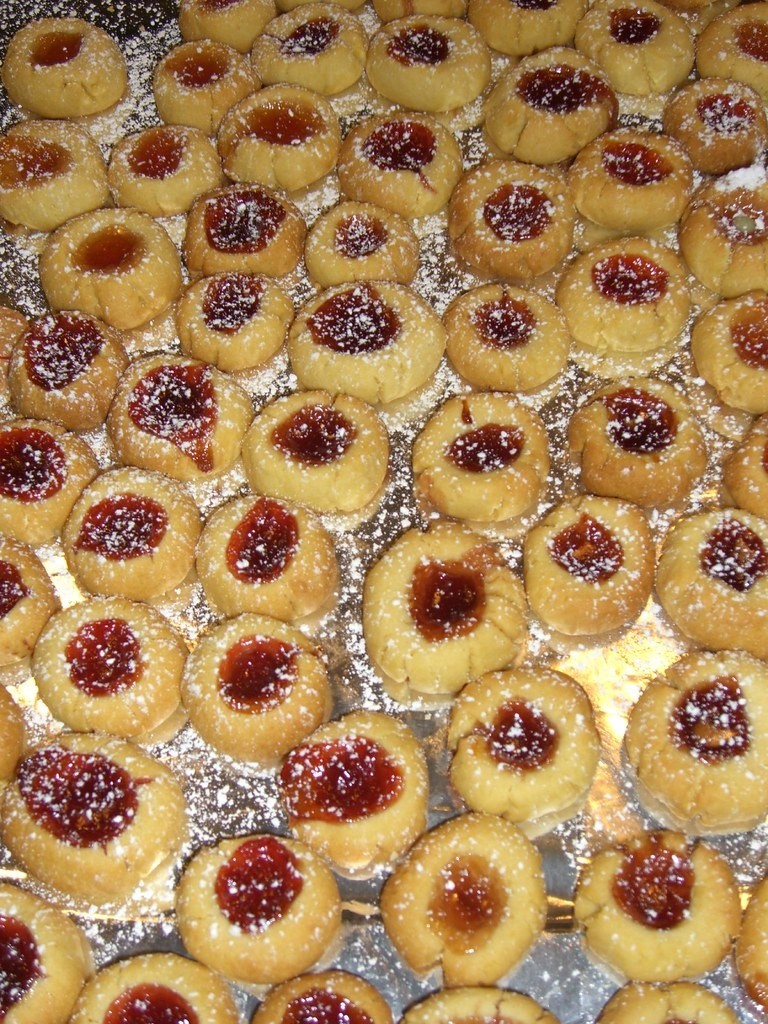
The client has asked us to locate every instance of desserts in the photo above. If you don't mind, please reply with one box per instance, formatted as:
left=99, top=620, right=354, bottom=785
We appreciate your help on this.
left=0, top=0, right=768, bottom=1024
